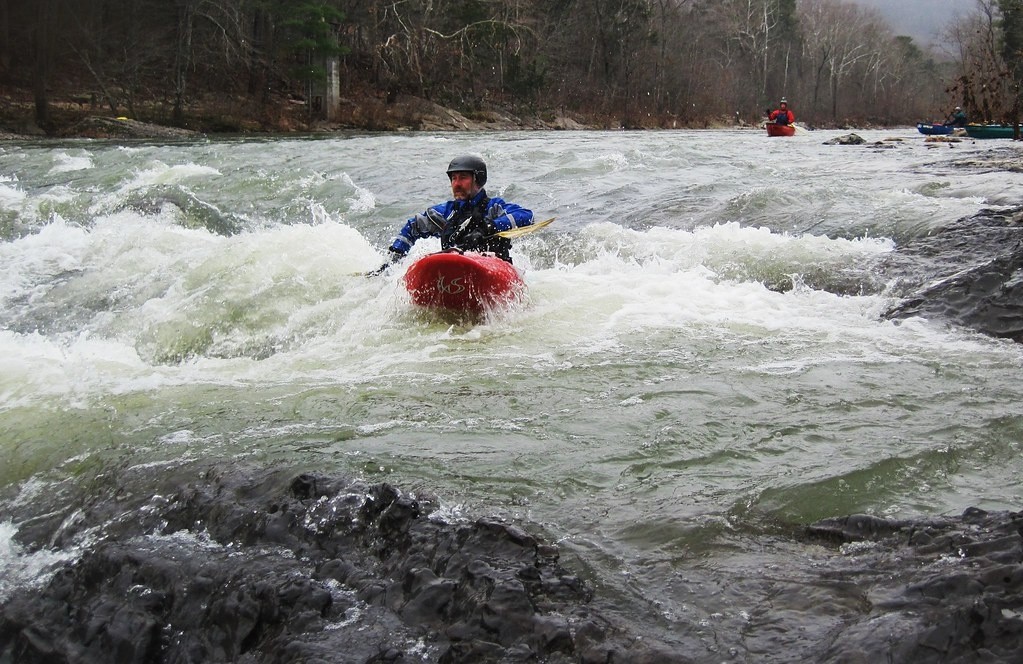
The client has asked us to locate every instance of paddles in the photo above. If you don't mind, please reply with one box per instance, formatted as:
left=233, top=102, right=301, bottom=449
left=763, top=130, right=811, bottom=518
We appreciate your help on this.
left=350, top=219, right=554, bottom=276
left=942, top=111, right=953, bottom=127
left=771, top=112, right=795, bottom=129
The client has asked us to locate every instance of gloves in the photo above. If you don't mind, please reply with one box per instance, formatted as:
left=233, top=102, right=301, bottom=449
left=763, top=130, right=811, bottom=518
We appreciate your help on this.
left=380, top=253, right=401, bottom=272
left=951, top=111, right=953, bottom=116
left=766, top=109, right=770, bottom=115
left=463, top=229, right=484, bottom=251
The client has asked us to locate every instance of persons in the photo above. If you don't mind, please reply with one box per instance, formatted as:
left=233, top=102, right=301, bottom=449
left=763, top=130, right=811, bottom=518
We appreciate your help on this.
left=366, top=154, right=535, bottom=277
left=766, top=97, right=794, bottom=126
left=944, top=107, right=965, bottom=127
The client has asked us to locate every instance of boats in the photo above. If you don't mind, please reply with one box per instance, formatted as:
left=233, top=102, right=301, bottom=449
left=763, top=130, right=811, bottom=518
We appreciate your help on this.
left=917, top=121, right=965, bottom=139
left=965, top=123, right=1023, bottom=139
left=767, top=122, right=795, bottom=137
left=402, top=246, right=526, bottom=325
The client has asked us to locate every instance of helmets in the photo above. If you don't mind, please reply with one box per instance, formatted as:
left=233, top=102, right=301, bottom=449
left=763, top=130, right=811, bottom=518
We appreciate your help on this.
left=780, top=97, right=787, bottom=106
left=954, top=107, right=961, bottom=110
left=446, top=156, right=488, bottom=186
